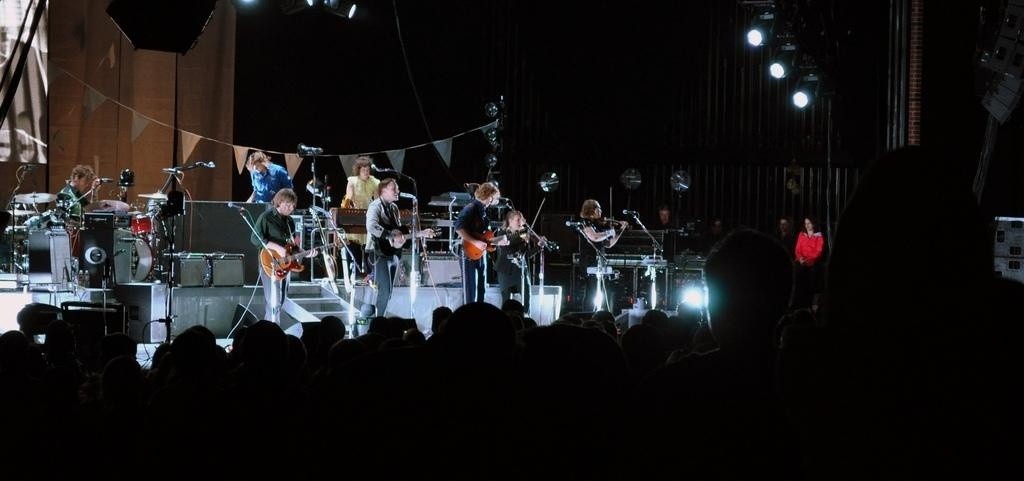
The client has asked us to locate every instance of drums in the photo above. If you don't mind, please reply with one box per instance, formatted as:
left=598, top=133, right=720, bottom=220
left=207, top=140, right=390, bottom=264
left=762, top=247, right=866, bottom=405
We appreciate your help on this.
left=132, top=236, right=155, bottom=281
left=6, top=227, right=30, bottom=239
left=130, top=212, right=153, bottom=233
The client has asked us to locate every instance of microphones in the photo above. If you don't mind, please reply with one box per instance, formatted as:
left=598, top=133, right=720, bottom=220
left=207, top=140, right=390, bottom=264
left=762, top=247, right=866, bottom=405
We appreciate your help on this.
left=623, top=209, right=637, bottom=215
left=100, top=178, right=115, bottom=183
left=500, top=197, right=509, bottom=203
left=198, top=160, right=216, bottom=169
left=63, top=264, right=73, bottom=292
left=566, top=221, right=583, bottom=227
left=399, top=192, right=414, bottom=199
left=227, top=201, right=246, bottom=212
left=371, top=163, right=385, bottom=173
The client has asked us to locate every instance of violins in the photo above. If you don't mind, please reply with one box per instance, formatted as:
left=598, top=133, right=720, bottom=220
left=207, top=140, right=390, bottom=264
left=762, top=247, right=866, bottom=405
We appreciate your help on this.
left=594, top=219, right=639, bottom=229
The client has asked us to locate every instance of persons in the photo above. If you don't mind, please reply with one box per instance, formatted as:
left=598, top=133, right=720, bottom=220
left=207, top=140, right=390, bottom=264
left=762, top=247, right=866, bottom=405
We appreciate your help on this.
left=251, top=187, right=318, bottom=328
left=794, top=216, right=825, bottom=308
left=778, top=216, right=793, bottom=245
left=58, top=163, right=97, bottom=219
left=702, top=217, right=725, bottom=256
left=564, top=198, right=628, bottom=311
left=454, top=182, right=511, bottom=304
left=492, top=210, right=548, bottom=318
left=1, top=300, right=1024, bottom=479
left=657, top=204, right=672, bottom=226
left=815, top=143, right=1023, bottom=334
left=245, top=150, right=294, bottom=203
left=701, top=228, right=794, bottom=359
left=463, top=181, right=479, bottom=195
left=359, top=178, right=436, bottom=323
left=342, top=155, right=381, bottom=286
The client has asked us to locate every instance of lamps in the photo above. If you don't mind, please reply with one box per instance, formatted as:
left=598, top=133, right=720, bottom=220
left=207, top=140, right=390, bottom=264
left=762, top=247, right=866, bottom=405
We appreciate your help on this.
left=279, top=0, right=358, bottom=20
left=741, top=0, right=824, bottom=111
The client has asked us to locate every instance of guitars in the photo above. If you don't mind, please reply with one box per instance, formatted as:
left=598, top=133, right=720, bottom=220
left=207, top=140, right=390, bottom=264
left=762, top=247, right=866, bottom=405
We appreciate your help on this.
left=260, top=239, right=351, bottom=281
left=463, top=228, right=520, bottom=261
left=379, top=225, right=442, bottom=256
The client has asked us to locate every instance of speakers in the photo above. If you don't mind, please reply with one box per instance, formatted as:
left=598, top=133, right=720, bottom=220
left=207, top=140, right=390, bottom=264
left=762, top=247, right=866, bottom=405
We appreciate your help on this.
left=616, top=310, right=642, bottom=337
left=79, top=228, right=114, bottom=288
left=120, top=283, right=173, bottom=343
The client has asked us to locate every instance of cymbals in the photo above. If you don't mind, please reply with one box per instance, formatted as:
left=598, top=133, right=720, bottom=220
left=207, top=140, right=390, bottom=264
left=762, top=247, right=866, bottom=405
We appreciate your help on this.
left=138, top=194, right=168, bottom=199
left=15, top=193, right=57, bottom=204
left=84, top=199, right=129, bottom=211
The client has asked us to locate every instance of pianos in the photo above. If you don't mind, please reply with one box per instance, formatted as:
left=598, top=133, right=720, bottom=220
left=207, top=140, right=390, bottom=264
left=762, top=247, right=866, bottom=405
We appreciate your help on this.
left=604, top=254, right=667, bottom=267
left=328, top=206, right=413, bottom=227
